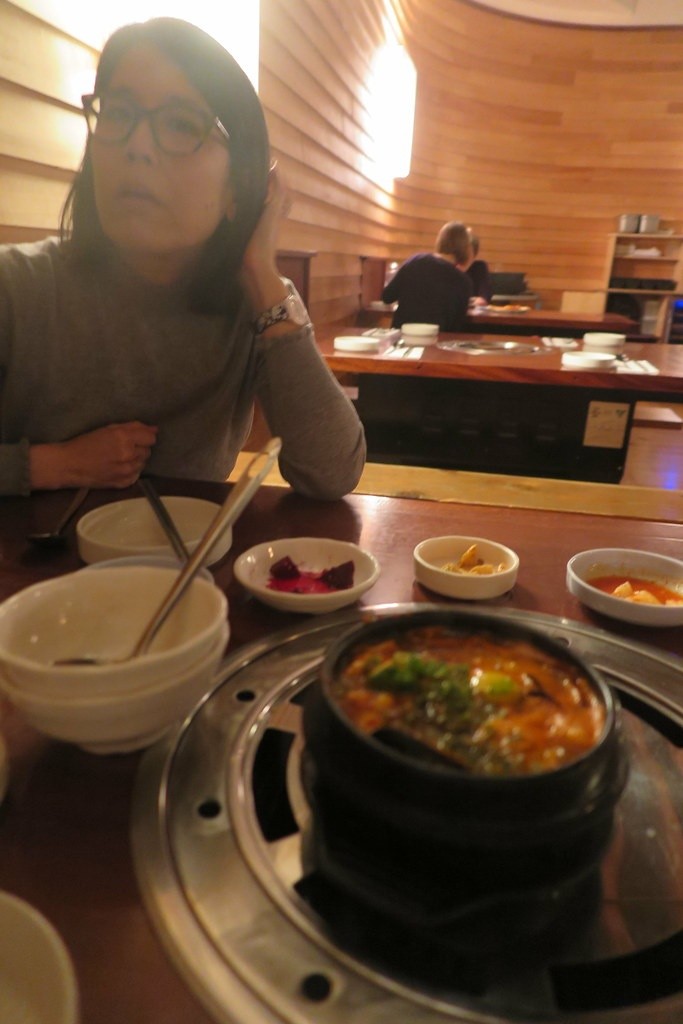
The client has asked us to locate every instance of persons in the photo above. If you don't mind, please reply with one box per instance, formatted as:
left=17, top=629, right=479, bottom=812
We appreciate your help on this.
left=0, top=17, right=366, bottom=498
left=381, top=221, right=493, bottom=329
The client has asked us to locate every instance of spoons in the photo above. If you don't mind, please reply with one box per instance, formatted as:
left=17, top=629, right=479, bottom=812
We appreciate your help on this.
left=617, top=354, right=632, bottom=370
left=384, top=339, right=404, bottom=356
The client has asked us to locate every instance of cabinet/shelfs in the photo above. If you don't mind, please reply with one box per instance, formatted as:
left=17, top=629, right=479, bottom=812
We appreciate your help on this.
left=596, top=232, right=683, bottom=342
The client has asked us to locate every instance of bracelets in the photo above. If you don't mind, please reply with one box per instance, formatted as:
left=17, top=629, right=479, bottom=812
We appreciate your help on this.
left=253, top=276, right=296, bottom=334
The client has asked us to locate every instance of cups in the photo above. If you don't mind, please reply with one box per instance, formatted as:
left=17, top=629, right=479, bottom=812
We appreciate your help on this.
left=619, top=213, right=637, bottom=233
left=638, top=214, right=659, bottom=233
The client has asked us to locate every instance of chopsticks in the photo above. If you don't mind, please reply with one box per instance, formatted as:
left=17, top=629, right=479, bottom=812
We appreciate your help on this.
left=136, top=470, right=194, bottom=565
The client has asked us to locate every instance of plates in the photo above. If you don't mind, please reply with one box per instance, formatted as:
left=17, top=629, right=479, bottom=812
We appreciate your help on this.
left=413, top=536, right=520, bottom=600
left=334, top=336, right=381, bottom=353
left=234, top=537, right=380, bottom=615
left=74, top=496, right=232, bottom=568
left=560, top=351, right=616, bottom=367
left=565, top=547, right=683, bottom=627
left=478, top=304, right=529, bottom=314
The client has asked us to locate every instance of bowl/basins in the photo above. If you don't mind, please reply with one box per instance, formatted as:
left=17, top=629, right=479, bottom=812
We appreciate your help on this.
left=302, top=610, right=631, bottom=887
left=1, top=567, right=230, bottom=758
left=401, top=323, right=439, bottom=345
left=583, top=332, right=626, bottom=356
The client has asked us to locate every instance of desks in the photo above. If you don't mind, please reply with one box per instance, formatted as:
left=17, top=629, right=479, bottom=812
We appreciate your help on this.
left=362, top=303, right=638, bottom=343
left=314, top=326, right=683, bottom=483
left=0, top=480, right=683, bottom=1024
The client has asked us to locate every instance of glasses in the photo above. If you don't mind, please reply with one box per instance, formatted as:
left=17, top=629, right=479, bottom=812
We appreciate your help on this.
left=82, top=93, right=232, bottom=156
